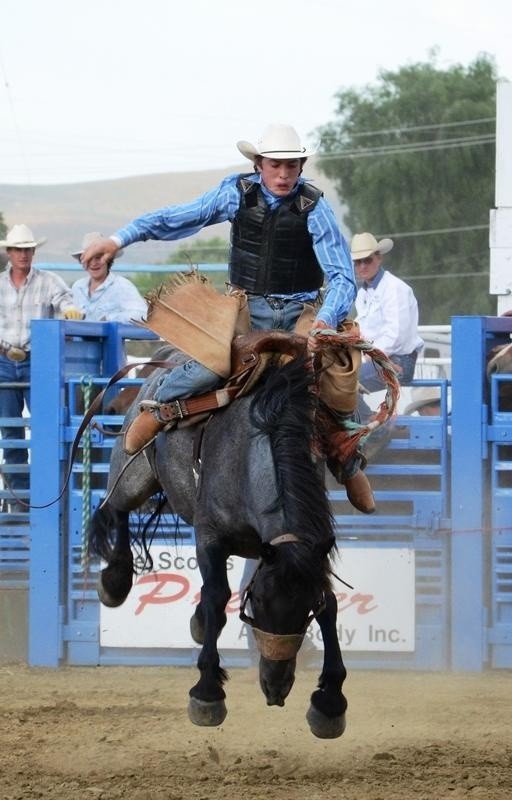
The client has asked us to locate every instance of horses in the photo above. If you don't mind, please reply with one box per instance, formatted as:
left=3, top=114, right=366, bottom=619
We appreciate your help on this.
left=79, top=343, right=357, bottom=741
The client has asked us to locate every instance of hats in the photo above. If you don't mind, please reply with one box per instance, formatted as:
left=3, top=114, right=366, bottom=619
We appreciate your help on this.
left=231, top=126, right=318, bottom=163
left=67, top=229, right=129, bottom=260
left=0, top=222, right=49, bottom=252
left=350, top=228, right=397, bottom=258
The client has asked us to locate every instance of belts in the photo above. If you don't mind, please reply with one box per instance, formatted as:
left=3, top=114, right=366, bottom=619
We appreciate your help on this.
left=0, top=348, right=10, bottom=356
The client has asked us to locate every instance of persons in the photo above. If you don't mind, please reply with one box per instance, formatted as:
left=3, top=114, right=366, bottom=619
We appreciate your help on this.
left=0, top=223, right=83, bottom=515
left=347, top=231, right=423, bottom=423
left=72, top=230, right=150, bottom=324
left=78, top=123, right=377, bottom=516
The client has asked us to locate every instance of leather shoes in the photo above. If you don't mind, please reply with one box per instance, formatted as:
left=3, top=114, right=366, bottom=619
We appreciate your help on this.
left=121, top=403, right=161, bottom=458
left=347, top=467, right=381, bottom=516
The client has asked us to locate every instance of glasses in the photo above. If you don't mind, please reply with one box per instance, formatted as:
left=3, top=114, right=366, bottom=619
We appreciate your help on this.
left=354, top=257, right=375, bottom=264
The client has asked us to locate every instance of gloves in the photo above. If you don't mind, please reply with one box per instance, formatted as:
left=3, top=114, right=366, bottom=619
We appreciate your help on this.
left=61, top=308, right=84, bottom=320
